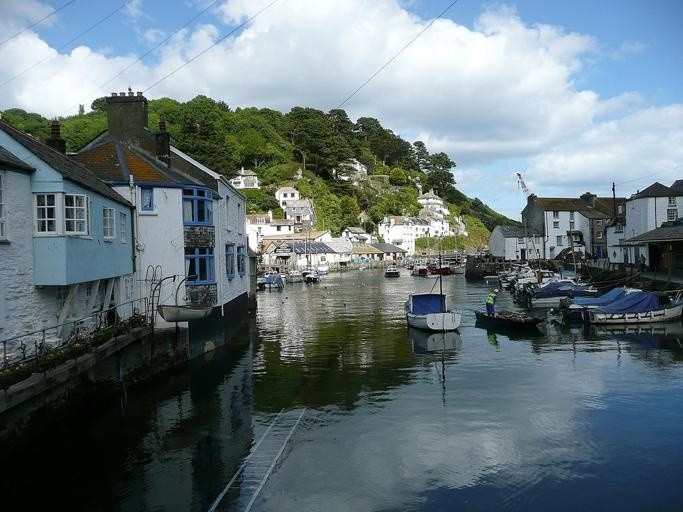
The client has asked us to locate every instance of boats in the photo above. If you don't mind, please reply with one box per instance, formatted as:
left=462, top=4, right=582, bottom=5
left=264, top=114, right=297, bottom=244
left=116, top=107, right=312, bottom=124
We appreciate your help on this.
left=474, top=320, right=544, bottom=345
left=481, top=219, right=682, bottom=323
left=400, top=291, right=459, bottom=332
left=379, top=234, right=467, bottom=279
left=406, top=324, right=460, bottom=359
left=257, top=227, right=329, bottom=289
left=584, top=322, right=682, bottom=348
left=474, top=308, right=545, bottom=327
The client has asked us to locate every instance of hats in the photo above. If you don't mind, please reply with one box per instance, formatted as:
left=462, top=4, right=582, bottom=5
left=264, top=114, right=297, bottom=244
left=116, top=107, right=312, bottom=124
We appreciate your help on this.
left=493, top=288, right=499, bottom=293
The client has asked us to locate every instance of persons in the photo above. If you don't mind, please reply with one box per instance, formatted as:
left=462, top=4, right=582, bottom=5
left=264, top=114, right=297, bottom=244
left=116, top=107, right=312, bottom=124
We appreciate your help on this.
left=486, top=289, right=498, bottom=317
left=637, top=255, right=646, bottom=272
